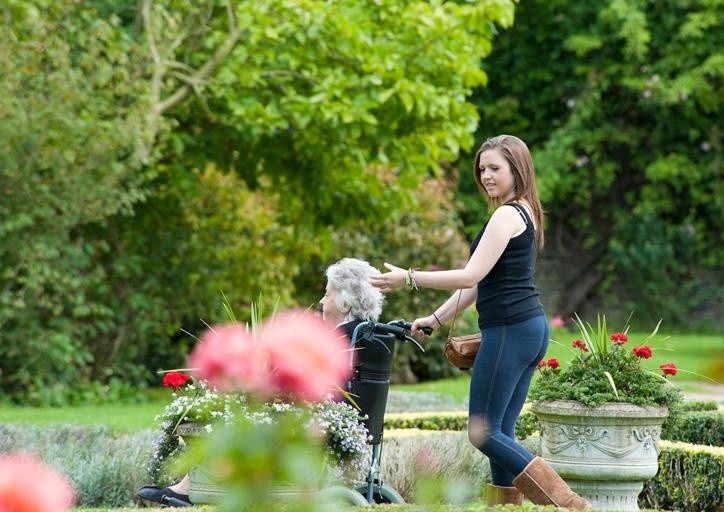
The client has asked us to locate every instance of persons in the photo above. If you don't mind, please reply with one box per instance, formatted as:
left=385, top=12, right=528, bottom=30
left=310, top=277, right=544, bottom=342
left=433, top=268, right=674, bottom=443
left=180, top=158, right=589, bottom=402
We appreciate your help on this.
left=138, top=256, right=386, bottom=508
left=368, top=134, right=594, bottom=512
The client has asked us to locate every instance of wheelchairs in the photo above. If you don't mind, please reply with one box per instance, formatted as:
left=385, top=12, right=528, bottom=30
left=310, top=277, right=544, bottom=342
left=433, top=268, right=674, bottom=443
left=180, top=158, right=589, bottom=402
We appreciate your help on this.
left=154, top=311, right=434, bottom=512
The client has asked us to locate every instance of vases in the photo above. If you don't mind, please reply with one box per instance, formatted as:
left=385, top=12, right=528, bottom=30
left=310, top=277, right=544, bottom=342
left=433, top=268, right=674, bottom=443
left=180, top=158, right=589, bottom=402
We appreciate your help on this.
left=532, top=401, right=669, bottom=511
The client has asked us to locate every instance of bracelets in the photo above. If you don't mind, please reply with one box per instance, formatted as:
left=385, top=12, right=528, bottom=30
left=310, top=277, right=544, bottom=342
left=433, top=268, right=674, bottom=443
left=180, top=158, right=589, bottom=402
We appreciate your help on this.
left=430, top=311, right=445, bottom=330
left=405, top=268, right=418, bottom=293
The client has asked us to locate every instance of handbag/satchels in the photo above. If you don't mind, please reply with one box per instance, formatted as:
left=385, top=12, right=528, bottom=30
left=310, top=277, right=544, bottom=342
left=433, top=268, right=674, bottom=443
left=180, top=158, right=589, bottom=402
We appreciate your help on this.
left=442, top=332, right=481, bottom=371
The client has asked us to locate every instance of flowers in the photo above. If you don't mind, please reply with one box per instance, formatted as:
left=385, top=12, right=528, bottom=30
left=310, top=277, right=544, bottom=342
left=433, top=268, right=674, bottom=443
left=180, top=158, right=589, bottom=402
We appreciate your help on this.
left=524, top=311, right=723, bottom=432
left=141, top=289, right=383, bottom=511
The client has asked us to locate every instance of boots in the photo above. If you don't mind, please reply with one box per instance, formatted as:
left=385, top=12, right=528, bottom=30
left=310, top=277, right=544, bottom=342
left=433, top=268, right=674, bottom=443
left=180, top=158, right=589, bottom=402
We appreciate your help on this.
left=482, top=481, right=523, bottom=507
left=511, top=452, right=590, bottom=511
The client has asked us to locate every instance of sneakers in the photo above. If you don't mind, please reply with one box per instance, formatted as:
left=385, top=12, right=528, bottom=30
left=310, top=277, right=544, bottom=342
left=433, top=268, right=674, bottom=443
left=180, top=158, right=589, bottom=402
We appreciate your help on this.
left=136, top=486, right=193, bottom=507
